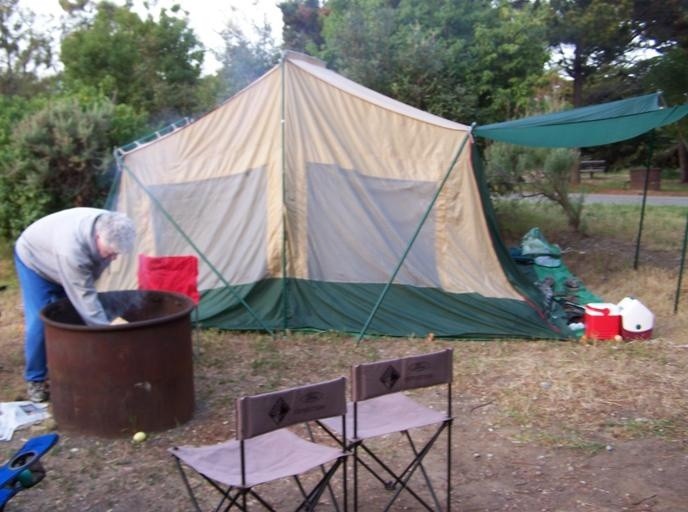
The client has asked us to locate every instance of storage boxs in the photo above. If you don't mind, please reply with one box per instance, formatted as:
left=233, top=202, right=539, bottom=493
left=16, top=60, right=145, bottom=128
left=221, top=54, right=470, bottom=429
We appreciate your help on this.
left=619, top=296, right=654, bottom=341
left=584, top=302, right=620, bottom=342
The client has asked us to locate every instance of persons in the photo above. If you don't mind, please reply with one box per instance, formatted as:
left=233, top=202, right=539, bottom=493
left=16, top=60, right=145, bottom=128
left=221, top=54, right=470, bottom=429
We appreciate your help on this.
left=13, top=206, right=137, bottom=404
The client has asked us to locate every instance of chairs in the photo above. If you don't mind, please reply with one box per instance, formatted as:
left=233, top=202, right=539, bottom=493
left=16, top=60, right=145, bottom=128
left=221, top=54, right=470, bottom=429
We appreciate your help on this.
left=166, top=376, right=348, bottom=512
left=317, top=347, right=453, bottom=512
left=137, top=254, right=201, bottom=362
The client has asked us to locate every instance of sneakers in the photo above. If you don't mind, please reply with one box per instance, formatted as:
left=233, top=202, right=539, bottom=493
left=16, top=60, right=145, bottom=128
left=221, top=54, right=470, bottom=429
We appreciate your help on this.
left=27, top=380, right=50, bottom=403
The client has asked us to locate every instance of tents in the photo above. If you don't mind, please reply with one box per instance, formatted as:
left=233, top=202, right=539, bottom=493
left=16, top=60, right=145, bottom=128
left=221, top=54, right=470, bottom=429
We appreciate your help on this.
left=104, top=51, right=687, bottom=342
